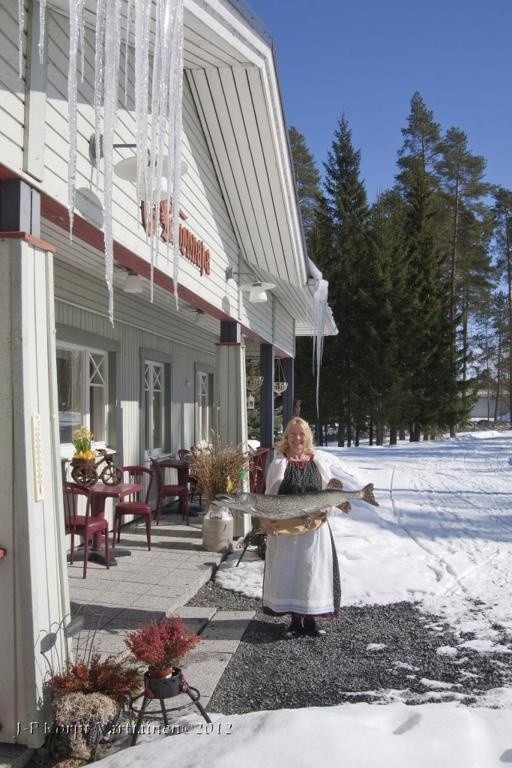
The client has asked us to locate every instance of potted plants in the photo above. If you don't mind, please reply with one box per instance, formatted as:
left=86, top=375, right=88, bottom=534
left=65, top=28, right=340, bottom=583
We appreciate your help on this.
left=123, top=611, right=209, bottom=745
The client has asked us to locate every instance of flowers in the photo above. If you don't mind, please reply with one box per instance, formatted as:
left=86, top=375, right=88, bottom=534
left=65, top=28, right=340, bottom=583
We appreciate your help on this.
left=70, top=426, right=96, bottom=458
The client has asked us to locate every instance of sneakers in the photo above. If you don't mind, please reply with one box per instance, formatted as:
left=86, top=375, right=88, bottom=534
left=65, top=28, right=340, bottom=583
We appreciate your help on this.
left=283, top=618, right=326, bottom=638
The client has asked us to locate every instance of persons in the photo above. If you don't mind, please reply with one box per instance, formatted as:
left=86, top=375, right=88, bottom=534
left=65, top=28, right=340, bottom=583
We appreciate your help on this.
left=262, top=417, right=341, bottom=640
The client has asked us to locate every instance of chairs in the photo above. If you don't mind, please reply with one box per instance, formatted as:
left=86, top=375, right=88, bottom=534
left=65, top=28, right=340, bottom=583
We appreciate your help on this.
left=60, top=447, right=272, bottom=579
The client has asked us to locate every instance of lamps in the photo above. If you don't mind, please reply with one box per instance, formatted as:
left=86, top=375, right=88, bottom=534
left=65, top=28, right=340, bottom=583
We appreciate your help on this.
left=91, top=135, right=189, bottom=205
left=225, top=266, right=277, bottom=303
